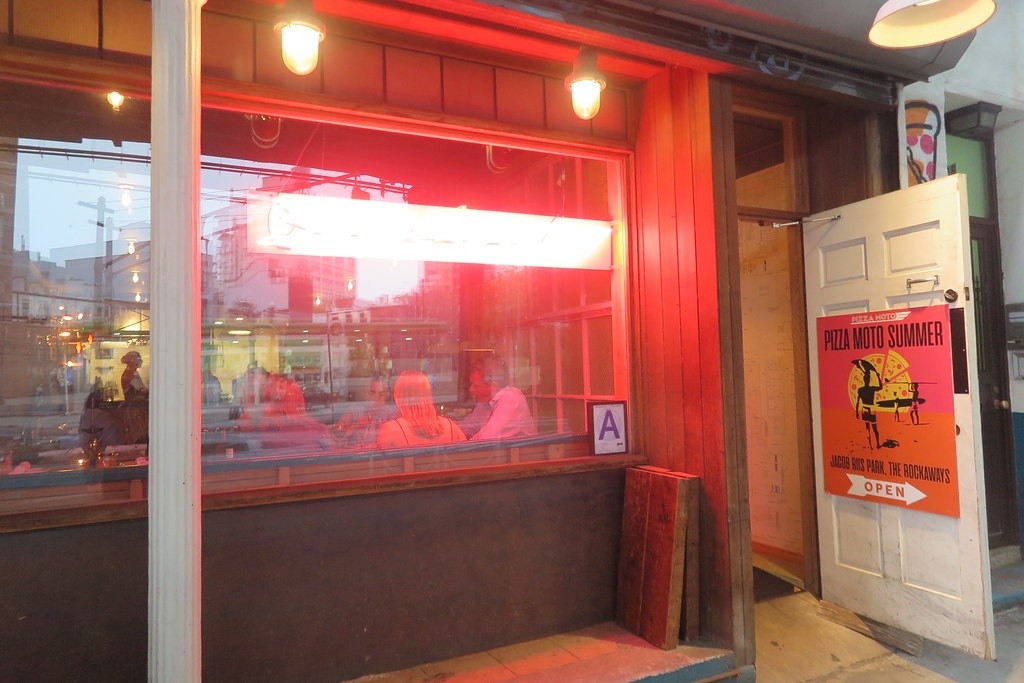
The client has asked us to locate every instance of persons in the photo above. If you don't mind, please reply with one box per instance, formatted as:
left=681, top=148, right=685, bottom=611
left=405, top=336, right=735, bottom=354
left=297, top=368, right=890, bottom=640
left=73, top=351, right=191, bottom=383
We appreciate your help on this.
left=377, top=370, right=467, bottom=448
left=203, top=367, right=336, bottom=452
left=78, top=368, right=149, bottom=500
left=122, top=351, right=149, bottom=401
left=333, top=374, right=399, bottom=446
left=457, top=357, right=537, bottom=440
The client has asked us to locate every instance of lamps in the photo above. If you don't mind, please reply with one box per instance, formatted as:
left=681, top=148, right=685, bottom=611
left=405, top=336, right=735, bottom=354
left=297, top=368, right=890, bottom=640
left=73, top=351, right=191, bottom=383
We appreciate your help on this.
left=272, top=0, right=327, bottom=78
left=866, top=0, right=998, bottom=48
left=564, top=45, right=607, bottom=120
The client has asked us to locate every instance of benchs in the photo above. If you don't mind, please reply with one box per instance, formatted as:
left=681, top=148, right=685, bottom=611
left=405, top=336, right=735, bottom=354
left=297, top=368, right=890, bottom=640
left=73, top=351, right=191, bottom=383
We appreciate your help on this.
left=0, top=433, right=586, bottom=517
